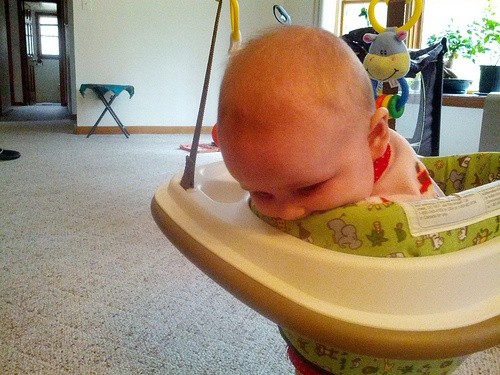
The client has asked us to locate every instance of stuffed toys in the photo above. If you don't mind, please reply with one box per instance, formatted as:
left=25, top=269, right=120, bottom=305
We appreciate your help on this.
left=228, top=0, right=243, bottom=55
left=363, top=0, right=424, bottom=120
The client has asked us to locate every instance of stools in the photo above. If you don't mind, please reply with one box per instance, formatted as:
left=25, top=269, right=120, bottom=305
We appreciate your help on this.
left=79, top=84, right=134, bottom=139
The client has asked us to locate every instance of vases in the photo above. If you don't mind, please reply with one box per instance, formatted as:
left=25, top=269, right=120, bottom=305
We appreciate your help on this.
left=442, top=79, right=473, bottom=94
left=473, top=64, right=500, bottom=97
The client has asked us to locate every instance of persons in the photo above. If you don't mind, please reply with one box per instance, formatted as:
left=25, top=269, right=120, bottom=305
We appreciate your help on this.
left=216, top=26, right=446, bottom=222
